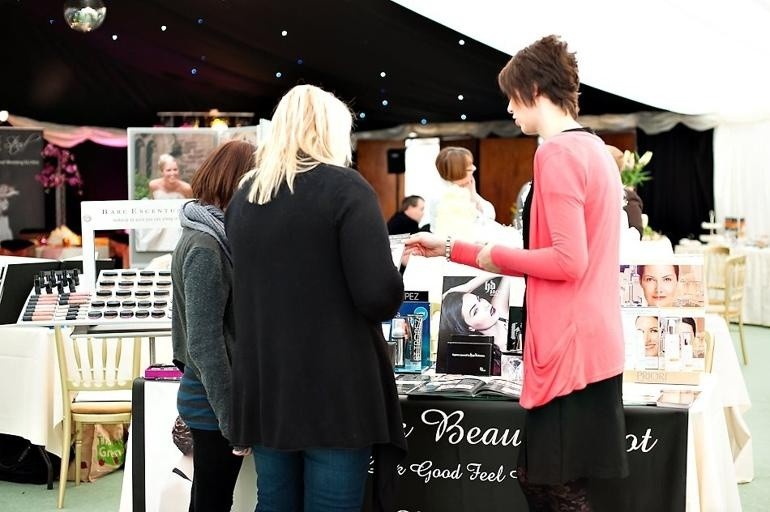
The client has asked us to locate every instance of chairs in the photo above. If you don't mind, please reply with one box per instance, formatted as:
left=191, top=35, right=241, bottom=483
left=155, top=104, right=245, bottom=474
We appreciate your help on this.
left=703, top=246, right=750, bottom=368
left=54, top=323, right=142, bottom=510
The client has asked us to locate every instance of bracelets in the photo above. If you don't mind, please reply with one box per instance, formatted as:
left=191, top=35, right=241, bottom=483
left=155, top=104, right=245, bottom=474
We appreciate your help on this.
left=445, top=237, right=452, bottom=263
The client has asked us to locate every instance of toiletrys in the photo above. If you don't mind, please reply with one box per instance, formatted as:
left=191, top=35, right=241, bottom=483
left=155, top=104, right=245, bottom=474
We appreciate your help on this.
left=382, top=312, right=426, bottom=372
left=22, top=267, right=173, bottom=322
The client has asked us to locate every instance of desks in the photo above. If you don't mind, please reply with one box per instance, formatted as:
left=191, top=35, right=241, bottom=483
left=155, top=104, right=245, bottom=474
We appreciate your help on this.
left=366, top=385, right=724, bottom=511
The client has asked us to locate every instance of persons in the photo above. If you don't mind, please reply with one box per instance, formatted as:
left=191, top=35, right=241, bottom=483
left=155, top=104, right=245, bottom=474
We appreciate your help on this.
left=434, top=145, right=497, bottom=235
left=170, top=139, right=257, bottom=512
left=402, top=32, right=634, bottom=507
left=148, top=153, right=192, bottom=199
left=639, top=265, right=680, bottom=308
left=635, top=315, right=658, bottom=358
left=224, top=81, right=409, bottom=512
left=440, top=272, right=509, bottom=350
left=387, top=193, right=429, bottom=235
left=606, top=143, right=646, bottom=241
left=682, top=318, right=696, bottom=345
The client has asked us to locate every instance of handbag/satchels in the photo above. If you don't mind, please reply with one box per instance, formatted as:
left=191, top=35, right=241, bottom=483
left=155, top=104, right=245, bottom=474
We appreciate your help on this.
left=68, top=423, right=124, bottom=483
left=0, top=433, right=60, bottom=485
